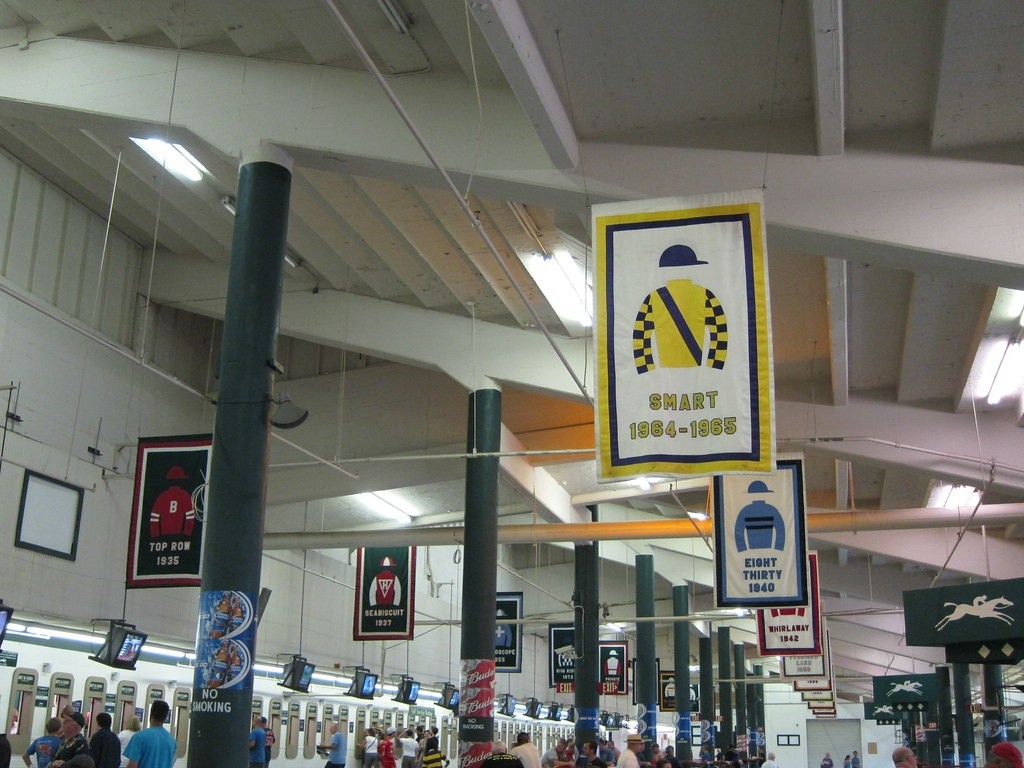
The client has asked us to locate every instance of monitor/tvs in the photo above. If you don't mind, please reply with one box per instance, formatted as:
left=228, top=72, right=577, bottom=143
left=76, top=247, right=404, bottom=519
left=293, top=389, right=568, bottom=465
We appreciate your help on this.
left=438, top=688, right=460, bottom=708
left=99, top=626, right=148, bottom=667
left=547, top=706, right=562, bottom=718
left=397, top=681, right=420, bottom=702
left=350, top=673, right=378, bottom=699
left=567, top=708, right=574, bottom=721
left=284, top=661, right=316, bottom=690
left=526, top=702, right=543, bottom=718
left=600, top=714, right=623, bottom=726
left=0, top=604, right=14, bottom=647
left=498, top=697, right=516, bottom=714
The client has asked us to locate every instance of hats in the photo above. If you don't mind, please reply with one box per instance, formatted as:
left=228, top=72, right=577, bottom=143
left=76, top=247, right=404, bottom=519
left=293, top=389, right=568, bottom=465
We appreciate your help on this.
left=64, top=712, right=84, bottom=729
left=990, top=742, right=1024, bottom=768
left=414, top=726, right=423, bottom=732
left=624, top=734, right=645, bottom=743
left=387, top=727, right=397, bottom=735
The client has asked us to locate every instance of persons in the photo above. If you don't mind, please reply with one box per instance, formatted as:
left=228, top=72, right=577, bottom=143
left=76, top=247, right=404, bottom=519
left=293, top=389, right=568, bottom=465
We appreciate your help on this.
left=480, top=741, right=525, bottom=768
left=822, top=752, right=833, bottom=768
left=583, top=741, right=608, bottom=768
left=248, top=716, right=275, bottom=768
left=0, top=707, right=18, bottom=768
left=510, top=732, right=576, bottom=768
left=701, top=743, right=781, bottom=768
left=122, top=700, right=178, bottom=768
left=357, top=725, right=450, bottom=768
left=892, top=747, right=918, bottom=768
left=649, top=743, right=684, bottom=768
left=319, top=724, right=347, bottom=768
left=843, top=755, right=852, bottom=768
left=982, top=742, right=1024, bottom=768
left=599, top=734, right=653, bottom=768
left=851, top=751, right=860, bottom=768
left=21, top=705, right=122, bottom=768
left=118, top=715, right=142, bottom=768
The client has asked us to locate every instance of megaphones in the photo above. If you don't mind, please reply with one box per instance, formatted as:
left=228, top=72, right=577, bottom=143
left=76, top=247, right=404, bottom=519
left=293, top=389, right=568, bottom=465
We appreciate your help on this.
left=270, top=391, right=309, bottom=429
left=603, top=602, right=610, bottom=618
